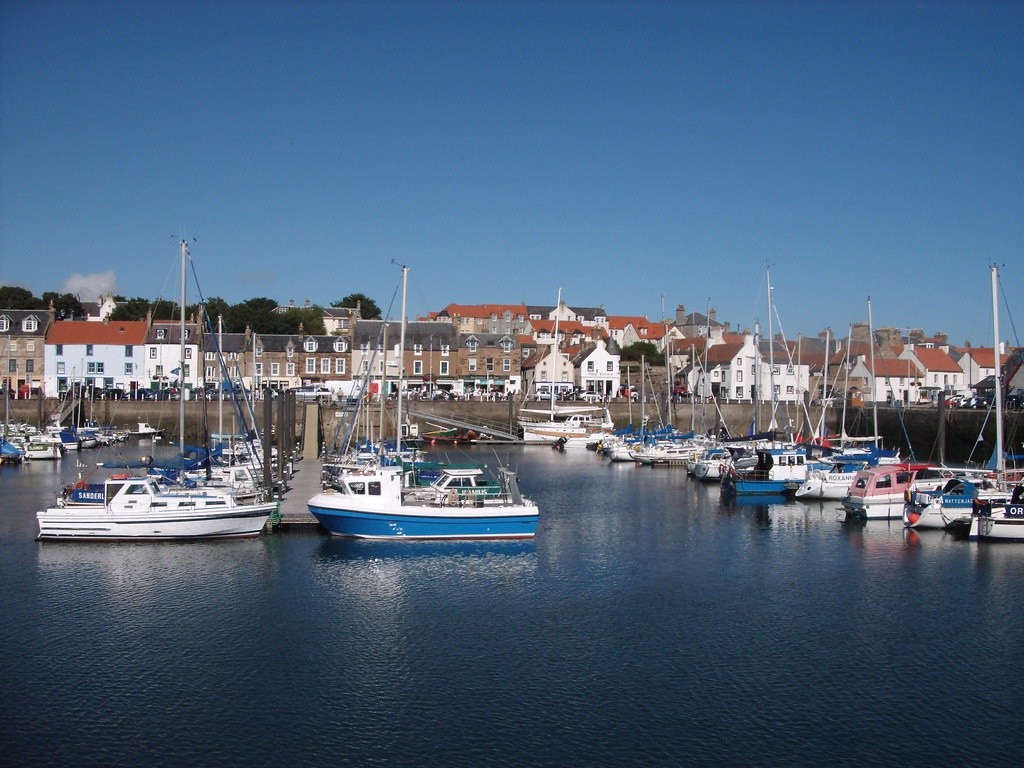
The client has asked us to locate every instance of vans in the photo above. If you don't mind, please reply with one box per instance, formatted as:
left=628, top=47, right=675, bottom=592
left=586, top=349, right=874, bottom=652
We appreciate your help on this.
left=289, top=387, right=332, bottom=399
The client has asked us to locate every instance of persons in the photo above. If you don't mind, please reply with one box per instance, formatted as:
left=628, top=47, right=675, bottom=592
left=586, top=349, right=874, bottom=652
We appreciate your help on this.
left=337, top=386, right=343, bottom=402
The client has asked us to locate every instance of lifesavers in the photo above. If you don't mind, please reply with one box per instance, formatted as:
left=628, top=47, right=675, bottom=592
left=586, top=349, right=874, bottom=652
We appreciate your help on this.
left=109, top=472, right=127, bottom=479
left=904, top=490, right=911, bottom=502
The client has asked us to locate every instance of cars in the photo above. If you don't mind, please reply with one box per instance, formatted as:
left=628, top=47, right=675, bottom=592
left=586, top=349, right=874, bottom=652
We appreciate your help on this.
left=1006, top=394, right=1023, bottom=409
left=976, top=398, right=997, bottom=408
left=672, top=384, right=689, bottom=397
left=145, top=389, right=171, bottom=402
left=95, top=388, right=123, bottom=399
left=531, top=390, right=560, bottom=400
left=945, top=394, right=965, bottom=407
left=225, top=387, right=251, bottom=402
left=122, top=388, right=148, bottom=400
left=204, top=389, right=220, bottom=401
left=967, top=396, right=982, bottom=409
left=172, top=390, right=197, bottom=400
left=576, top=390, right=602, bottom=401
left=956, top=397, right=969, bottom=409
left=392, top=388, right=416, bottom=399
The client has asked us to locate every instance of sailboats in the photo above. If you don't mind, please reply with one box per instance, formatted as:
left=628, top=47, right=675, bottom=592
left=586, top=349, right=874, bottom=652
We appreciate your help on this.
left=1, top=238, right=1024, bottom=543
